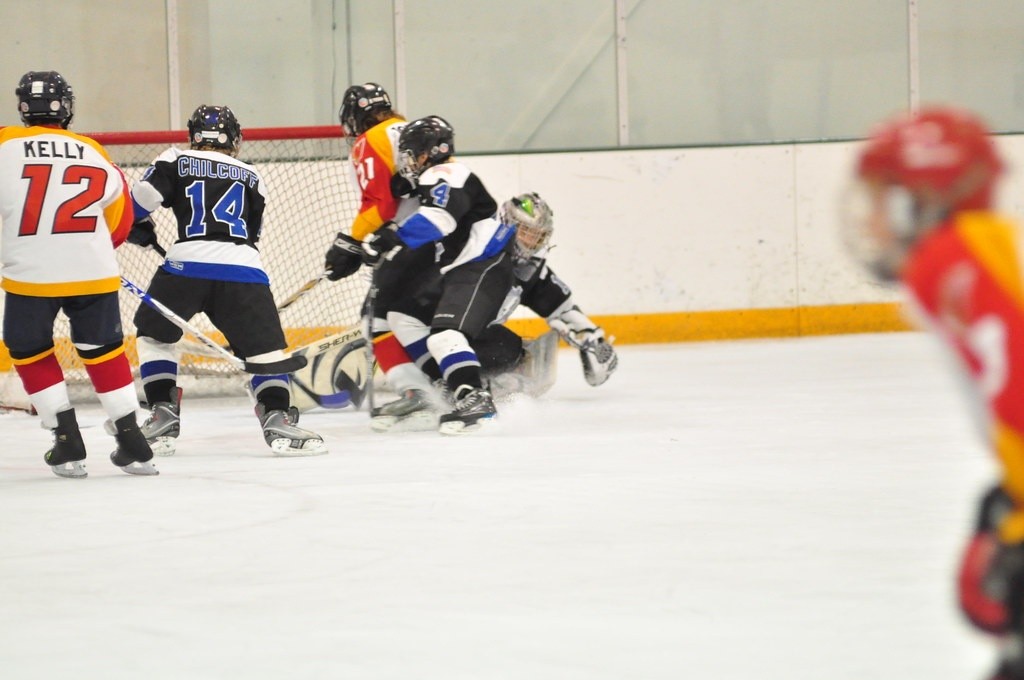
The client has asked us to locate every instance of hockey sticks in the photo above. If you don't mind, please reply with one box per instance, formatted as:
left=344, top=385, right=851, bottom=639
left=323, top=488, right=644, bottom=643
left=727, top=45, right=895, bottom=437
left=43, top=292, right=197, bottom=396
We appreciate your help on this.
left=178, top=268, right=334, bottom=359
left=363, top=283, right=427, bottom=417
left=171, top=324, right=366, bottom=379
left=151, top=242, right=352, bottom=410
left=120, top=274, right=310, bottom=378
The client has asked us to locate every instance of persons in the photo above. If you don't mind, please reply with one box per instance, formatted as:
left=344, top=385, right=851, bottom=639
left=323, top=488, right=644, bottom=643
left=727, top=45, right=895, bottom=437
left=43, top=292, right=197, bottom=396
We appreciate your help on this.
left=839, top=105, right=1024, bottom=680
left=126, top=103, right=324, bottom=453
left=0, top=71, right=154, bottom=466
left=324, top=83, right=618, bottom=430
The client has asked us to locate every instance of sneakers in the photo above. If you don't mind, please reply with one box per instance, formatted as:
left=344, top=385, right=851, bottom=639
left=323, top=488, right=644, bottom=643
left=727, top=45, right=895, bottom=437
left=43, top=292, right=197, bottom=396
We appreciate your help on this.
left=104, top=411, right=159, bottom=475
left=44, top=407, right=88, bottom=479
left=255, top=403, right=324, bottom=455
left=581, top=338, right=617, bottom=386
left=370, top=388, right=440, bottom=431
left=140, top=387, right=182, bottom=455
left=439, top=379, right=497, bottom=437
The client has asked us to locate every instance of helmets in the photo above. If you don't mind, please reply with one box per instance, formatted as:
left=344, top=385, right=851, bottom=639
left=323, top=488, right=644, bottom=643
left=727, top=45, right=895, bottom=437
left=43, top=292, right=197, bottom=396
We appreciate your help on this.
left=840, top=108, right=1003, bottom=282
left=188, top=104, right=241, bottom=151
left=339, top=82, right=391, bottom=141
left=16, top=69, right=74, bottom=129
left=398, top=115, right=454, bottom=179
left=498, top=193, right=554, bottom=265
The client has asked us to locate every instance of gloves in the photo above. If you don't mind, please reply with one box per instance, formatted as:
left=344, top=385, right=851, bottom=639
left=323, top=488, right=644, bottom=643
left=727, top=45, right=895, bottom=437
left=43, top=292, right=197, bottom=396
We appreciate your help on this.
left=390, top=167, right=419, bottom=200
left=325, top=231, right=363, bottom=281
left=958, top=488, right=1024, bottom=631
left=361, top=220, right=404, bottom=266
left=127, top=221, right=156, bottom=247
